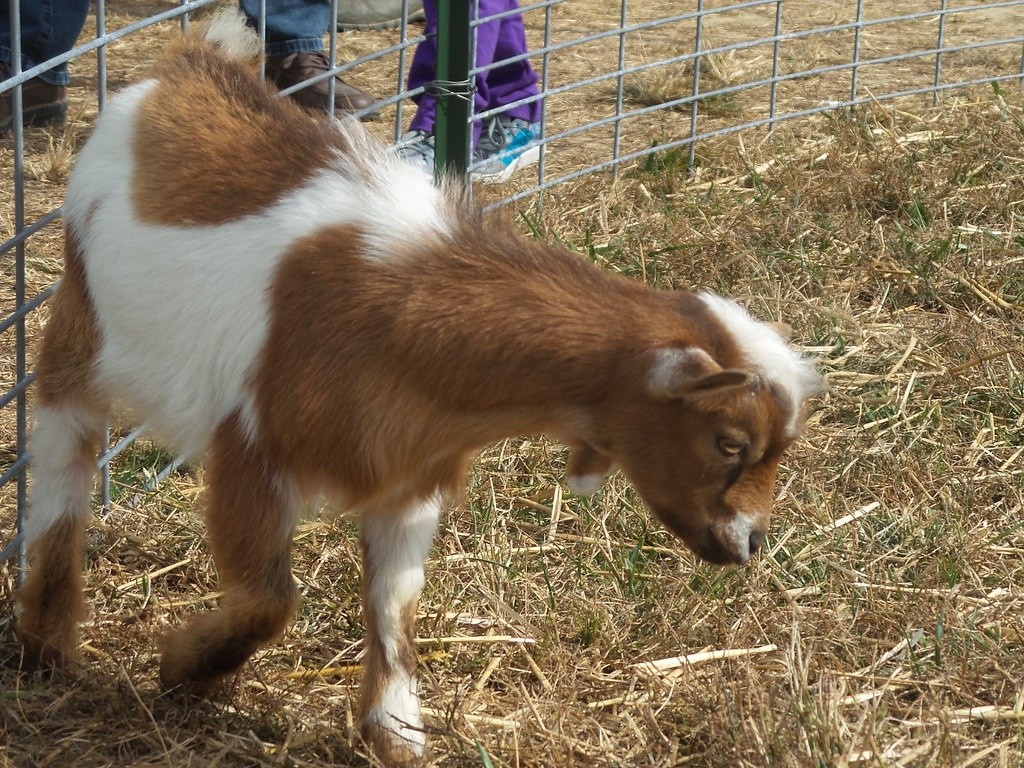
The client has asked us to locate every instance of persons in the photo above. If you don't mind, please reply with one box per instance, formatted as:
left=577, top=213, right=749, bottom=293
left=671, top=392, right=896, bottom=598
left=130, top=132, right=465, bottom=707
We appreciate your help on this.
left=1, top=0, right=385, bottom=130
left=391, top=0, right=545, bottom=183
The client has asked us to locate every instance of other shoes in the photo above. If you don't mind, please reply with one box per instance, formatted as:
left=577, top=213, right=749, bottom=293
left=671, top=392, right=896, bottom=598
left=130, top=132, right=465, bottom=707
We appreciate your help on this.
left=0, top=58, right=68, bottom=137
left=331, top=0, right=426, bottom=30
left=264, top=50, right=379, bottom=118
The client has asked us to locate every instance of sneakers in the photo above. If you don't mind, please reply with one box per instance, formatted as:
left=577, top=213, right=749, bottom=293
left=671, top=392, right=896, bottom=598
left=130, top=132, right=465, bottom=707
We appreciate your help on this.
left=471, top=112, right=547, bottom=184
left=392, top=129, right=434, bottom=177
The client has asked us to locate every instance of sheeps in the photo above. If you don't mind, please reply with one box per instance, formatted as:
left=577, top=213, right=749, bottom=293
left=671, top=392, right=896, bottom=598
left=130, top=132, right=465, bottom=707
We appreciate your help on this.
left=10, top=1, right=834, bottom=768
